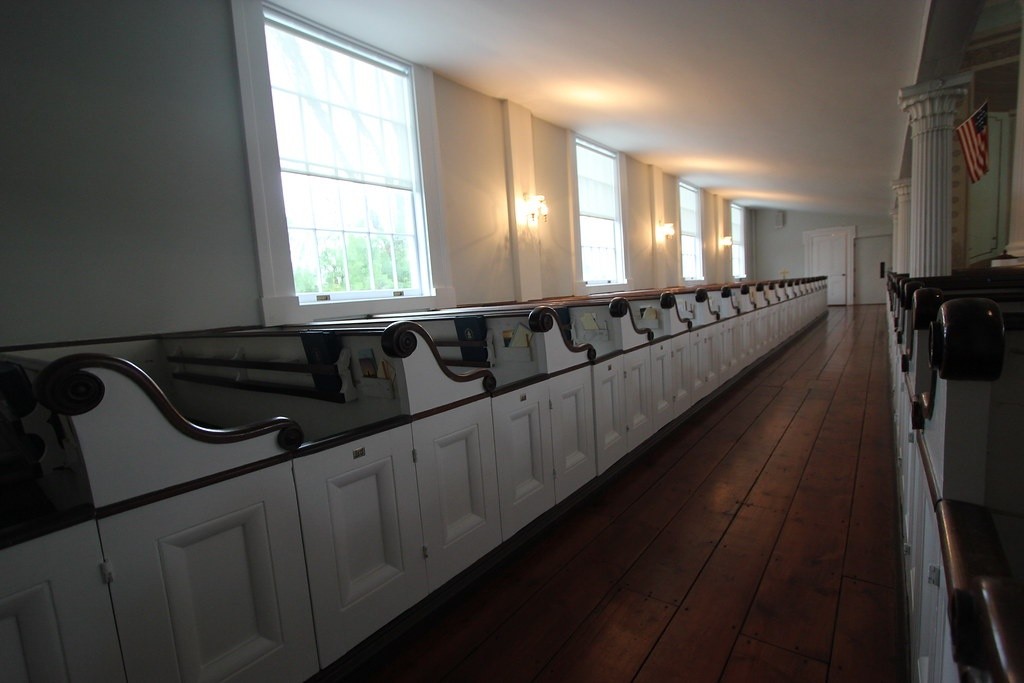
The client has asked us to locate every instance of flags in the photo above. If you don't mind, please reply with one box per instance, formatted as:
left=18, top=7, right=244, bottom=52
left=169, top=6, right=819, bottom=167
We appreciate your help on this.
left=958, top=102, right=989, bottom=184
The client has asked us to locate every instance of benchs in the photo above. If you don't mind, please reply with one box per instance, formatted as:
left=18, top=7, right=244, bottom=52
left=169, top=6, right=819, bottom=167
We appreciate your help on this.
left=0, top=272, right=1024, bottom=683
